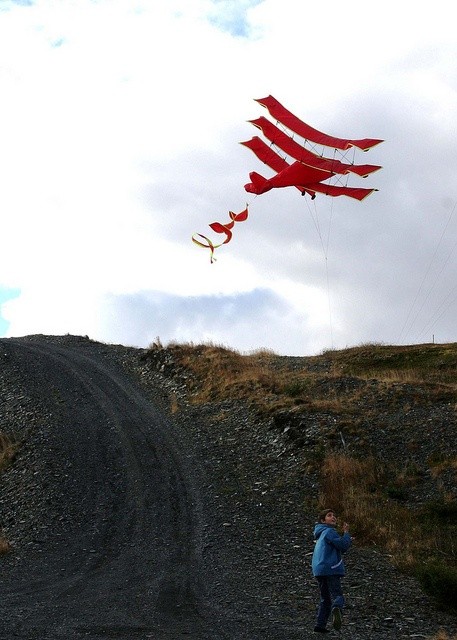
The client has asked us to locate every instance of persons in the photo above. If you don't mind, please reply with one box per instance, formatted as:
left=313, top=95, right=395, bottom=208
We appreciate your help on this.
left=311, top=509, right=351, bottom=637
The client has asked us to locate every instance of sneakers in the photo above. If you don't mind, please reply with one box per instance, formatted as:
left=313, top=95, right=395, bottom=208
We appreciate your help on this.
left=314, top=625, right=330, bottom=634
left=331, top=606, right=342, bottom=632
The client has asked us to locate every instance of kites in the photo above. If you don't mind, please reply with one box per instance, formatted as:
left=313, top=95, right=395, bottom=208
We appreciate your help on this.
left=191, top=93, right=384, bottom=265
